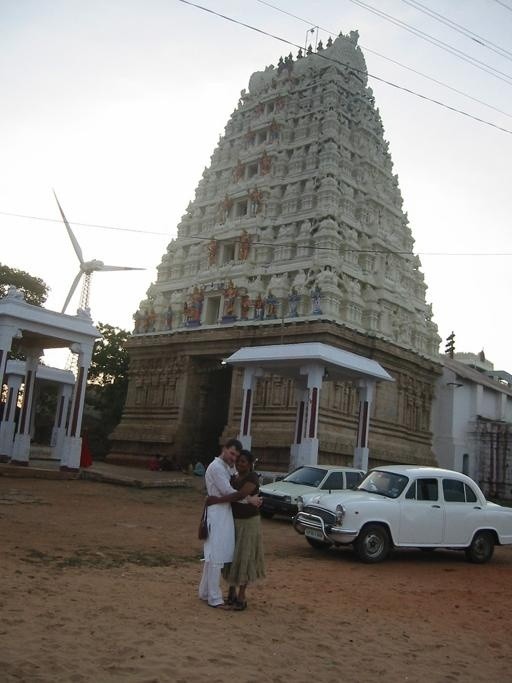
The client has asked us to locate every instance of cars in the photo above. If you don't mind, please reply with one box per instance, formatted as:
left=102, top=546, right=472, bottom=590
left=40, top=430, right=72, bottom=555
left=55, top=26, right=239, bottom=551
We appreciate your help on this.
left=291, top=464, right=512, bottom=564
left=260, top=461, right=376, bottom=521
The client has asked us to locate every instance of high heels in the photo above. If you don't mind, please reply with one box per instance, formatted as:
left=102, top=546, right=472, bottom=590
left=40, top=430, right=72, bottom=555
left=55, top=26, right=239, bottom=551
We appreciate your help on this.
left=233, top=599, right=247, bottom=611
left=228, top=593, right=237, bottom=605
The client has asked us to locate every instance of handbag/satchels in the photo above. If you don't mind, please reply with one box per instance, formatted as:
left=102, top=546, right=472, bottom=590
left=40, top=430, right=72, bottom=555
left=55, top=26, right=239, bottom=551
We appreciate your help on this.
left=198, top=504, right=207, bottom=540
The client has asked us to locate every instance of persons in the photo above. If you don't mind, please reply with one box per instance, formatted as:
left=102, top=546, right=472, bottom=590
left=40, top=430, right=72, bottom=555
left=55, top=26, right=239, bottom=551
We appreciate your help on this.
left=204, top=449, right=266, bottom=610
left=197, top=439, right=263, bottom=608
